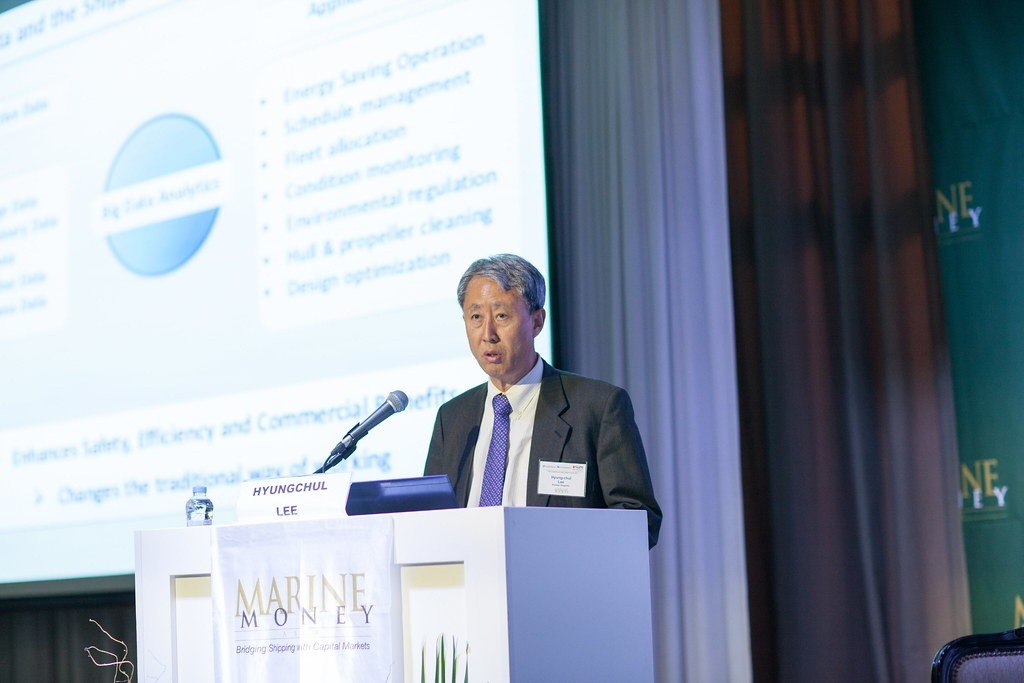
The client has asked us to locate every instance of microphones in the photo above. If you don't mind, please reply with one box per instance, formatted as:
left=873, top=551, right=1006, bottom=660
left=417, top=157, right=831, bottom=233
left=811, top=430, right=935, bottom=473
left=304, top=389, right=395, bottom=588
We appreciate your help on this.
left=330, top=390, right=410, bottom=460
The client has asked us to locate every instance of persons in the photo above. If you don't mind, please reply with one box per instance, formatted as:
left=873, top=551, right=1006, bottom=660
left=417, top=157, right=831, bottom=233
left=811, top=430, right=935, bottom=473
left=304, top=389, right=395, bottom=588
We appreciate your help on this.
left=423, top=254, right=661, bottom=549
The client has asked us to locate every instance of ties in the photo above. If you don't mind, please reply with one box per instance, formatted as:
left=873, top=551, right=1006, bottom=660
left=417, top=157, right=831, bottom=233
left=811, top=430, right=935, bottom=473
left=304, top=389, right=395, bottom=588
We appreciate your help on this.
left=479, top=394, right=513, bottom=506
